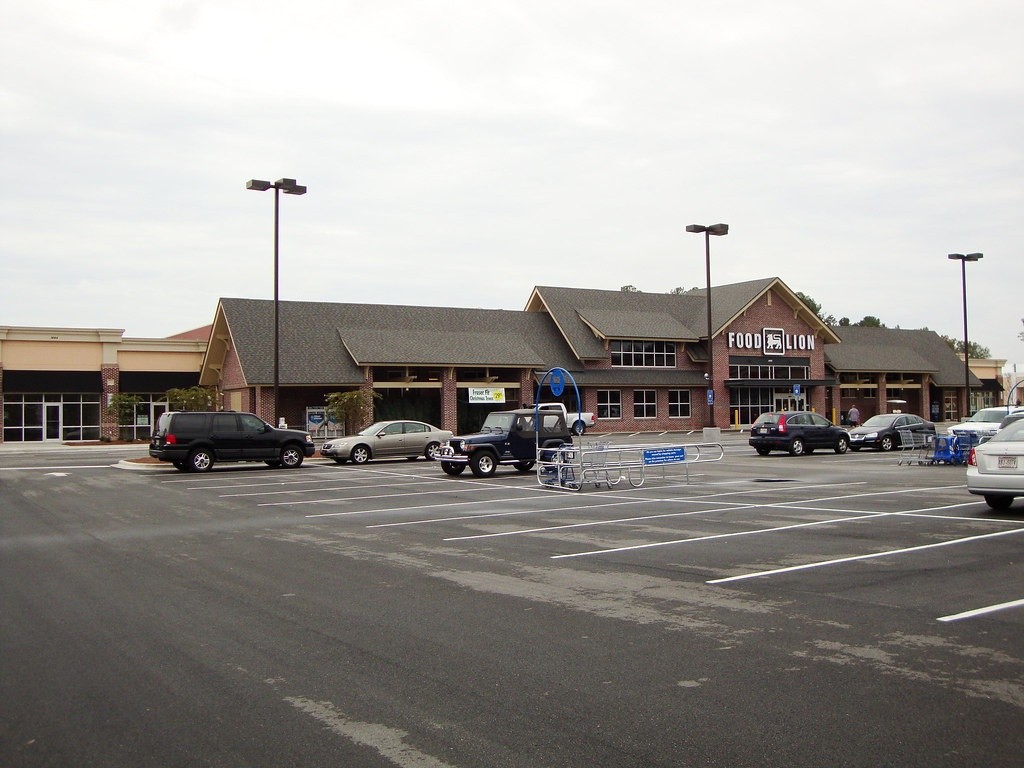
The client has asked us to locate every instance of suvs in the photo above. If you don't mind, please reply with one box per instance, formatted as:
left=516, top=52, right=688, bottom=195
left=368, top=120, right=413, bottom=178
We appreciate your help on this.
left=433, top=408, right=574, bottom=478
left=748, top=411, right=850, bottom=457
left=148, top=410, right=316, bottom=470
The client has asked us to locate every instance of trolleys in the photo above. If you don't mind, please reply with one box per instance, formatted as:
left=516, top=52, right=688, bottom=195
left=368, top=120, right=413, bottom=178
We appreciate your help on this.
left=896, top=427, right=935, bottom=467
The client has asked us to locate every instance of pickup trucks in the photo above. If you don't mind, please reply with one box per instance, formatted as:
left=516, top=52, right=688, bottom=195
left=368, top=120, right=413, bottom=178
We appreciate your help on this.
left=516, top=403, right=597, bottom=436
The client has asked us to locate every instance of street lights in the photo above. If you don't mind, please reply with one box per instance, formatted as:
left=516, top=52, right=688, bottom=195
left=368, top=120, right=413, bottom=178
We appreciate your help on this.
left=246, top=178, right=309, bottom=429
left=947, top=251, right=984, bottom=417
left=685, top=223, right=732, bottom=448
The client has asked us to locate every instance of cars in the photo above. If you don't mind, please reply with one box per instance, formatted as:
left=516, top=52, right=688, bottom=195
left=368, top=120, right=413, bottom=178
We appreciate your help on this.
left=319, top=420, right=454, bottom=463
left=948, top=406, right=1023, bottom=448
left=996, top=409, right=1024, bottom=435
left=965, top=418, right=1024, bottom=510
left=848, top=413, right=936, bottom=452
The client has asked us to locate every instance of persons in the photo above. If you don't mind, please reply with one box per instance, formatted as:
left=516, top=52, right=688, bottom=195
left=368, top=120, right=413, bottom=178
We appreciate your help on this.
left=847, top=405, right=859, bottom=429
